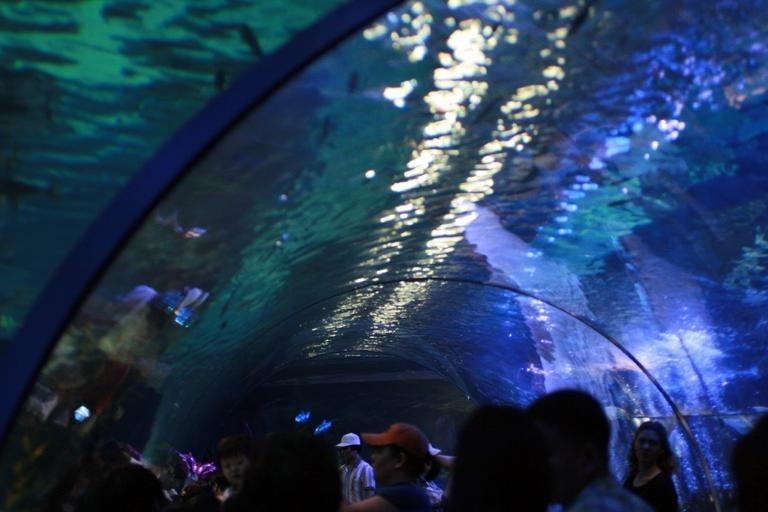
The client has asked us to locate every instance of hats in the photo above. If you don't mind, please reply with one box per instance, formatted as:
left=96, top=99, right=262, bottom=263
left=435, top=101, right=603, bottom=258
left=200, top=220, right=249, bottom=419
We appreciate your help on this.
left=360, top=422, right=428, bottom=461
left=335, top=432, right=360, bottom=447
left=428, top=443, right=442, bottom=457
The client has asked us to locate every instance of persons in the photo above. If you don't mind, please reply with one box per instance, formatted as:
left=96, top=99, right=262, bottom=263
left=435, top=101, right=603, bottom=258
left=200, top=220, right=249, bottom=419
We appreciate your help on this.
left=40, top=310, right=767, bottom=512
